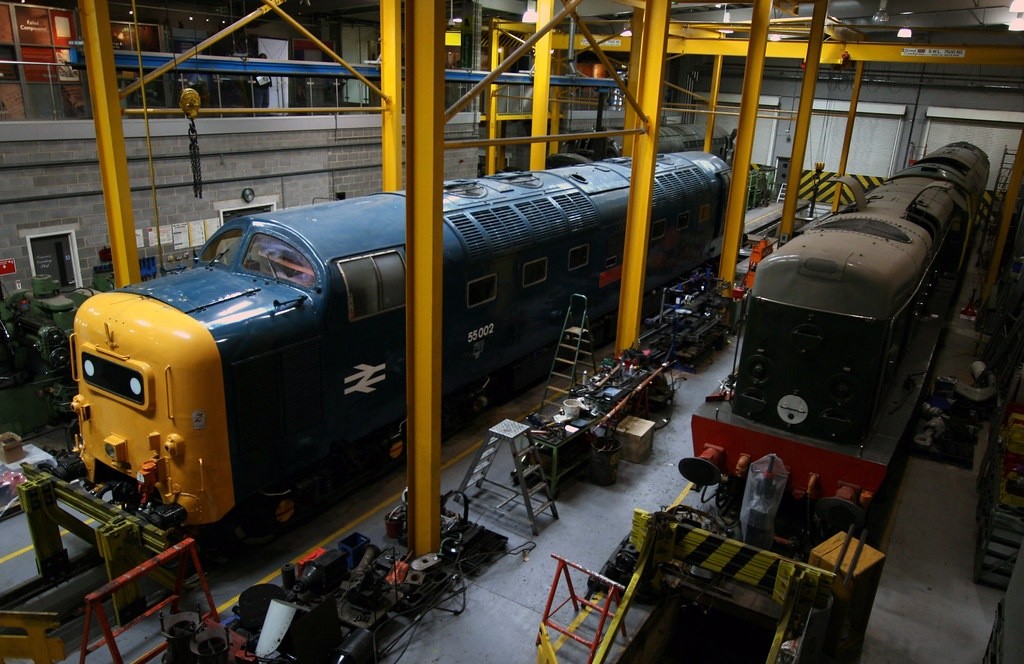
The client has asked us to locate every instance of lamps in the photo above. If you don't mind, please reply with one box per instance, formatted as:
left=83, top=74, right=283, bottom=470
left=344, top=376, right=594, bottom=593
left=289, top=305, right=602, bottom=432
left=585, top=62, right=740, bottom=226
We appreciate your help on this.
left=620, top=14, right=632, bottom=38
left=522, top=0, right=538, bottom=25
left=897, top=14, right=913, bottom=39
left=871, top=0, right=890, bottom=24
left=718, top=5, right=735, bottom=35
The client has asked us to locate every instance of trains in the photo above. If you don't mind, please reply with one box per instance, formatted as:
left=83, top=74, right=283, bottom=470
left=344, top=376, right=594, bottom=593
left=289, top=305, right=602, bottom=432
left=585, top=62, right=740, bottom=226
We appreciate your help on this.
left=40, top=149, right=732, bottom=574
left=675, top=140, right=991, bottom=564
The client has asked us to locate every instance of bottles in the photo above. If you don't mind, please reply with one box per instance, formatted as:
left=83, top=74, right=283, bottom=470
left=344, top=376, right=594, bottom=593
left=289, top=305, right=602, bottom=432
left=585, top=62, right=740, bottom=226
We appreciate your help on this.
left=583, top=370, right=587, bottom=384
left=629, top=363, right=638, bottom=375
left=621, top=361, right=626, bottom=376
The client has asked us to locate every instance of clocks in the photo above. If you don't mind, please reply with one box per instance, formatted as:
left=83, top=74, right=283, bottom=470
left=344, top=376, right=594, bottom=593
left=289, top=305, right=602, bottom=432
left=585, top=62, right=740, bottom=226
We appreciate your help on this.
left=242, top=188, right=255, bottom=203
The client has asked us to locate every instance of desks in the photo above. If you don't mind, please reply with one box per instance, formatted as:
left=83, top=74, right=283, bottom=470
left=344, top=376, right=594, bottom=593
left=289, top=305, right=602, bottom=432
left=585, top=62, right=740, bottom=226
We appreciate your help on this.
left=514, top=359, right=649, bottom=500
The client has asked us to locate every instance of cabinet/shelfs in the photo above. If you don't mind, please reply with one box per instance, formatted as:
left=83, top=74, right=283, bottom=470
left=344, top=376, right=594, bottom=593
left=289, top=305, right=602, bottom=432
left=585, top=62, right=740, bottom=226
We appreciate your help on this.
left=808, top=531, right=886, bottom=664
left=973, top=365, right=1024, bottom=592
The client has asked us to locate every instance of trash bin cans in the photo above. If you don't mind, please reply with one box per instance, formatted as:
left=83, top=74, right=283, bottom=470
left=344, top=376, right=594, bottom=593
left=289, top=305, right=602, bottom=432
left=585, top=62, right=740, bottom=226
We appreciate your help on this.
left=589, top=435, right=624, bottom=487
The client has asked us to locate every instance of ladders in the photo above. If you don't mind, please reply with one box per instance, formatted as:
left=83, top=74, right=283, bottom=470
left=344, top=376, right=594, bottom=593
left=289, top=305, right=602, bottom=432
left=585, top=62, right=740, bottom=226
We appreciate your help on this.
left=537, top=291, right=597, bottom=415
left=452, top=417, right=560, bottom=537
left=976, top=147, right=1016, bottom=254
left=775, top=182, right=788, bottom=204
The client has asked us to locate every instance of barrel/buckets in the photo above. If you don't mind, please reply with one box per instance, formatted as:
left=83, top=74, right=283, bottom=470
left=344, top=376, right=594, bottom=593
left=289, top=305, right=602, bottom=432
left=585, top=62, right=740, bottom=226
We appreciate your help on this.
left=590, top=436, right=622, bottom=486
left=558, top=60, right=606, bottom=109
left=562, top=399, right=580, bottom=417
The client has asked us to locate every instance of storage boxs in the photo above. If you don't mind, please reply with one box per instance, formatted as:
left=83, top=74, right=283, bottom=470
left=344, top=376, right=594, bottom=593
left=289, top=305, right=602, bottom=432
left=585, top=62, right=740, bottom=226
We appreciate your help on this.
left=339, top=532, right=371, bottom=570
left=0, top=431, right=23, bottom=464
left=929, top=376, right=965, bottom=408
left=616, top=415, right=656, bottom=465
